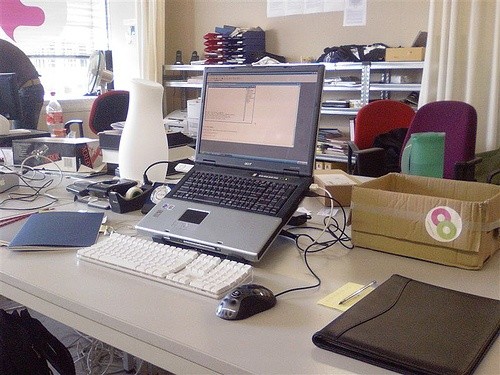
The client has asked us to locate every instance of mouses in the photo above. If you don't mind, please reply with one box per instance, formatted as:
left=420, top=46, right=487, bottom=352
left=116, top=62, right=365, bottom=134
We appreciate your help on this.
left=215, top=285, right=274, bottom=321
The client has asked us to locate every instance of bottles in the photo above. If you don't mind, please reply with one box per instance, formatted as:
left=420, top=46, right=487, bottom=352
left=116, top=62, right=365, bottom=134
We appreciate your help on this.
left=47, top=91, right=64, bottom=138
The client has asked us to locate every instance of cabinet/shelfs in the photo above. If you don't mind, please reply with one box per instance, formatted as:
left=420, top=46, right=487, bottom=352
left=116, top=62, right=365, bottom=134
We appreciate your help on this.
left=161, top=61, right=425, bottom=164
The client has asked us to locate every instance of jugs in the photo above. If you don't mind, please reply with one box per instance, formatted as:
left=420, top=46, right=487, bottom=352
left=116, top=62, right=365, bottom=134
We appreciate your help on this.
left=400, top=132, right=446, bottom=179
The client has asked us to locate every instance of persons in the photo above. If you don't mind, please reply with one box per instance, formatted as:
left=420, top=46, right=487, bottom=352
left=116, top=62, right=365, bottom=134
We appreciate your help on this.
left=0, top=38, right=44, bottom=130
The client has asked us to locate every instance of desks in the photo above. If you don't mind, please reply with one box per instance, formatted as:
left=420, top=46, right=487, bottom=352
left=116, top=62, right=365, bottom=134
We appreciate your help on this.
left=0, top=145, right=500, bottom=375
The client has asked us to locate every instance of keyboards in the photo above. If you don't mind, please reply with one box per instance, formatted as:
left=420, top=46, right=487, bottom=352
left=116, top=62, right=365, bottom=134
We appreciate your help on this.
left=75, top=233, right=254, bottom=299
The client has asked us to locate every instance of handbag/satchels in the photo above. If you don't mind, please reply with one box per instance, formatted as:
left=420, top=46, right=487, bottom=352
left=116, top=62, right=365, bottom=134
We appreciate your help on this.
left=373, top=127, right=406, bottom=172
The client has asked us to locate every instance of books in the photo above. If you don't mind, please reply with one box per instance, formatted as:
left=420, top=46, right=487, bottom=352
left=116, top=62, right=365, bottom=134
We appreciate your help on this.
left=314, top=128, right=350, bottom=156
left=321, top=99, right=349, bottom=108
left=402, top=91, right=419, bottom=111
left=9, top=212, right=105, bottom=251
left=98, top=121, right=193, bottom=178
left=323, top=75, right=363, bottom=85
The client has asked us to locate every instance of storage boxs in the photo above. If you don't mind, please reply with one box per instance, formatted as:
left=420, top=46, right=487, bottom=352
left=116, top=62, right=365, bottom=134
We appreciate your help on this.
left=313, top=169, right=362, bottom=207
left=351, top=171, right=500, bottom=271
left=385, top=47, right=426, bottom=62
left=12, top=137, right=103, bottom=173
left=97, top=129, right=124, bottom=151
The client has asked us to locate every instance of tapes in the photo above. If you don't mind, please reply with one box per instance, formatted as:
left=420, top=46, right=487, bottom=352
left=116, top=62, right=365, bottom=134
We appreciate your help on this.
left=126, top=186, right=143, bottom=199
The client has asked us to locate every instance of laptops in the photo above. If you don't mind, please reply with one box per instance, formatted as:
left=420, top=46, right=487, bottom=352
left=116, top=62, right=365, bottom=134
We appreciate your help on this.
left=135, top=62, right=326, bottom=264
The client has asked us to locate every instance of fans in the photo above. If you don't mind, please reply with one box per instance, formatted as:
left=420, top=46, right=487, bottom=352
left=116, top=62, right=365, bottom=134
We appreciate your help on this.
left=85, top=49, right=114, bottom=95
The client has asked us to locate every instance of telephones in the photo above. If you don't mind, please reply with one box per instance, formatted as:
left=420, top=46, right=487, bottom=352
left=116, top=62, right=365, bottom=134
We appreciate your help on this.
left=176, top=50, right=182, bottom=64
left=189, top=51, right=199, bottom=65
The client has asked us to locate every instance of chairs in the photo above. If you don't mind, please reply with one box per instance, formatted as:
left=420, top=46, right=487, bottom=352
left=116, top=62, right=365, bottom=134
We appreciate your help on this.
left=64, top=91, right=130, bottom=162
left=354, top=99, right=483, bottom=182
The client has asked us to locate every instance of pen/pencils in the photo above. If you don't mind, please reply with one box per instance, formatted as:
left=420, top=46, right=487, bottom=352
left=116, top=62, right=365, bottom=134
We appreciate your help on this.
left=0, top=212, right=39, bottom=226
left=338, top=280, right=376, bottom=304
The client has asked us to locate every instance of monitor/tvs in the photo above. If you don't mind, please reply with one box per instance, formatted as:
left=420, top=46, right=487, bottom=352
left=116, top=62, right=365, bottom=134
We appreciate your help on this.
left=0, top=73, right=24, bottom=121
